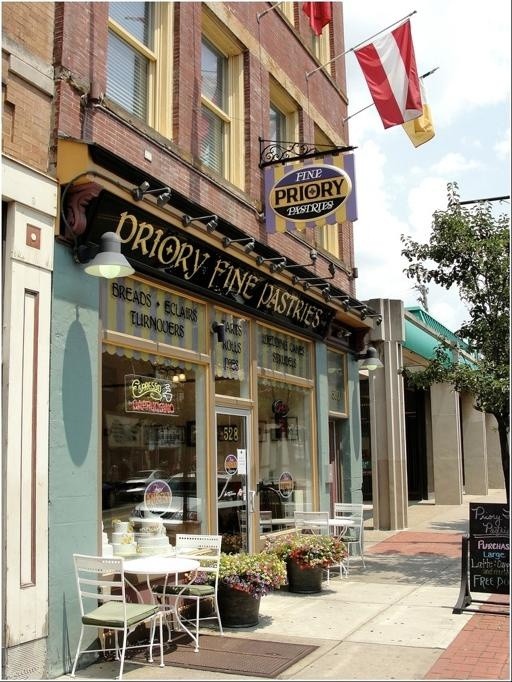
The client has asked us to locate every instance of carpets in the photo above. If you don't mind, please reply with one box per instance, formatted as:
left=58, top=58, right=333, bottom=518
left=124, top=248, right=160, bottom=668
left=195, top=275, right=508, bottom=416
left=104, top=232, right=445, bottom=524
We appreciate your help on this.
left=115, top=629, right=320, bottom=679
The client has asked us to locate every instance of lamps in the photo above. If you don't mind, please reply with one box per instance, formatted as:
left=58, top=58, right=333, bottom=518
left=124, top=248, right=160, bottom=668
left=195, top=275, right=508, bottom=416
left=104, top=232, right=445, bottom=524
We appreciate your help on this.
left=222, top=234, right=256, bottom=256
left=181, top=211, right=218, bottom=235
left=286, top=248, right=318, bottom=268
left=73, top=230, right=141, bottom=284
left=303, top=281, right=330, bottom=298
left=325, top=293, right=351, bottom=311
left=292, top=262, right=336, bottom=285
left=131, top=182, right=173, bottom=207
left=365, top=313, right=382, bottom=327
left=351, top=343, right=386, bottom=371
left=350, top=303, right=368, bottom=321
left=255, top=253, right=288, bottom=272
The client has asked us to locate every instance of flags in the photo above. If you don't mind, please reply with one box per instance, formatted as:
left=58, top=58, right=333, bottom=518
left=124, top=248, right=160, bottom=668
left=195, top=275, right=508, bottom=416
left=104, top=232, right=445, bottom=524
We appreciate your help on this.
left=401, top=79, right=434, bottom=148
left=354, top=19, right=422, bottom=129
left=302, top=2, right=331, bottom=36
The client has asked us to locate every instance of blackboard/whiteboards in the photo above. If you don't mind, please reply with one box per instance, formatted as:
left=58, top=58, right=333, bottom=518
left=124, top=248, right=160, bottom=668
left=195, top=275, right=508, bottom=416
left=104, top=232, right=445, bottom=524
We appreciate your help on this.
left=469, top=502, right=510, bottom=595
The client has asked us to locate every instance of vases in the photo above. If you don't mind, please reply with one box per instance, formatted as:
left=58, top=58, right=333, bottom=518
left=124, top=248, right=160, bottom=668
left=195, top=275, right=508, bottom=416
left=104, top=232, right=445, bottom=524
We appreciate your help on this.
left=213, top=587, right=260, bottom=628
left=284, top=558, right=324, bottom=594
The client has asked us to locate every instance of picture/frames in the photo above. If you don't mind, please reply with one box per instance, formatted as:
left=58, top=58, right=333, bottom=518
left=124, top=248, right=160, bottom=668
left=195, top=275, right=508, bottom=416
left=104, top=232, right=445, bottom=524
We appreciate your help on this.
left=143, top=424, right=164, bottom=445
left=167, top=426, right=185, bottom=442
left=256, top=420, right=268, bottom=443
left=186, top=420, right=196, bottom=448
left=267, top=415, right=300, bottom=442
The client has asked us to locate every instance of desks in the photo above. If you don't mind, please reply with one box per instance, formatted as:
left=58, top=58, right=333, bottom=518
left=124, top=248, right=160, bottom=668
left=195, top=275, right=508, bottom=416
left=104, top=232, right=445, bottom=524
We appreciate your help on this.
left=303, top=519, right=354, bottom=578
left=258, top=518, right=295, bottom=534
left=117, top=556, right=200, bottom=661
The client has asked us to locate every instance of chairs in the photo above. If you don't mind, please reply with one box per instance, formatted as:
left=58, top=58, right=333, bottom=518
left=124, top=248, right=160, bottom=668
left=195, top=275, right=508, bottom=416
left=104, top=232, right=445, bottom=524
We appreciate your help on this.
left=151, top=534, right=227, bottom=652
left=292, top=510, right=343, bottom=585
left=330, top=502, right=366, bottom=578
left=237, top=508, right=273, bottom=551
left=282, top=502, right=314, bottom=530
left=65, top=551, right=166, bottom=682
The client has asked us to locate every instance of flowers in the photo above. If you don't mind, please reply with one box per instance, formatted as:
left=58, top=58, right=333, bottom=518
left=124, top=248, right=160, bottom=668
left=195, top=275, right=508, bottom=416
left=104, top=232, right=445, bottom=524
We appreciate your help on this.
left=183, top=548, right=290, bottom=598
left=264, top=532, right=351, bottom=569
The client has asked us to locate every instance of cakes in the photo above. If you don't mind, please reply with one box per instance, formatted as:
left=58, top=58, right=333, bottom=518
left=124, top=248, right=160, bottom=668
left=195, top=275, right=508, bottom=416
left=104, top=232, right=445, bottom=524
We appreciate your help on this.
left=102, top=509, right=170, bottom=557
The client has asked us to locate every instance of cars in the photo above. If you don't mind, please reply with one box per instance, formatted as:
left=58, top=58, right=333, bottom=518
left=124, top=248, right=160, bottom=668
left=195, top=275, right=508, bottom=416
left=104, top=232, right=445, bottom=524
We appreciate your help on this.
left=122, top=469, right=170, bottom=497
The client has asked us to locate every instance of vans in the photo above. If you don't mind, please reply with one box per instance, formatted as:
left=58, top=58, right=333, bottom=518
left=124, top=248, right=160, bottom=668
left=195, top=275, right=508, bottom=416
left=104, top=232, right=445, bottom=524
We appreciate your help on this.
left=126, top=471, right=242, bottom=536
